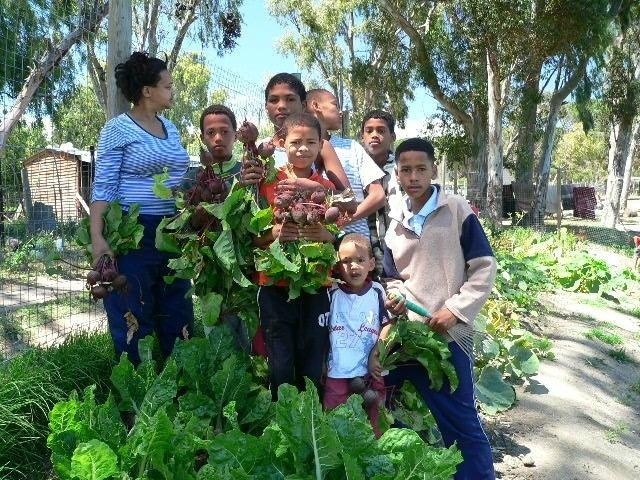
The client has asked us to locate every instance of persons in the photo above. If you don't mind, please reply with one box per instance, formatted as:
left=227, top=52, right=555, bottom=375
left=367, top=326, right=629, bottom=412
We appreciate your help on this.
left=199, top=73, right=496, bottom=479
left=89, top=51, right=194, bottom=367
